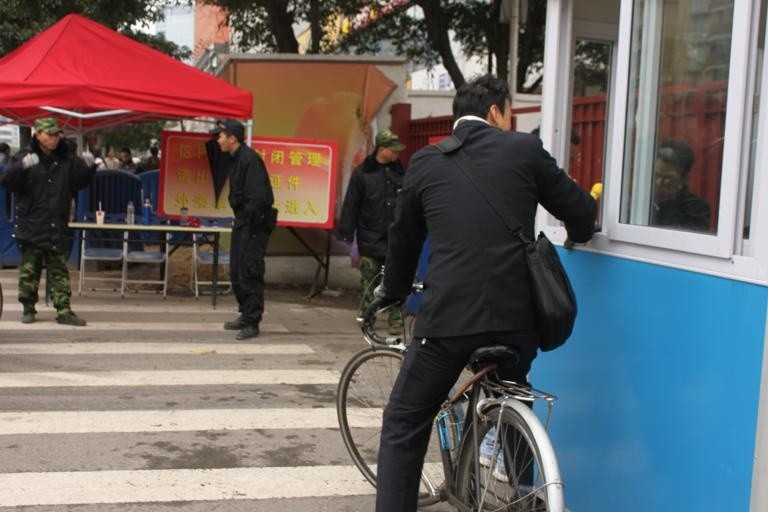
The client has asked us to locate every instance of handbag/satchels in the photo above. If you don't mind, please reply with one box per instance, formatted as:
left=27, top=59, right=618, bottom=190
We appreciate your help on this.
left=523, top=232, right=578, bottom=351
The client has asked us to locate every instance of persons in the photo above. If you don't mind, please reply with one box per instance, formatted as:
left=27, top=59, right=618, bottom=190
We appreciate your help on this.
left=0, top=117, right=92, bottom=327
left=373, top=74, right=597, bottom=511
left=209, top=119, right=275, bottom=340
left=650, top=139, right=711, bottom=234
left=1, top=141, right=161, bottom=179
left=337, top=130, right=407, bottom=335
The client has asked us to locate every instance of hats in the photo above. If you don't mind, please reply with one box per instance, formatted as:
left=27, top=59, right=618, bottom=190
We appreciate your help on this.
left=374, top=129, right=408, bottom=152
left=208, top=119, right=245, bottom=137
left=657, top=140, right=696, bottom=170
left=33, top=117, right=65, bottom=136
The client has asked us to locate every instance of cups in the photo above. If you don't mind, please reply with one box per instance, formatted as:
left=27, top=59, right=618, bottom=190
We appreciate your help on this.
left=95, top=211, right=106, bottom=226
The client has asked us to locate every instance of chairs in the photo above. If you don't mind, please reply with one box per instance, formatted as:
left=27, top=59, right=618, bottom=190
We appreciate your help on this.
left=189, top=215, right=232, bottom=299
left=77, top=211, right=125, bottom=300
left=124, top=213, right=171, bottom=299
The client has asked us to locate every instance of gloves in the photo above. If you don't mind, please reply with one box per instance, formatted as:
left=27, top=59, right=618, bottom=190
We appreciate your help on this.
left=22, top=152, right=39, bottom=169
left=82, top=150, right=95, bottom=167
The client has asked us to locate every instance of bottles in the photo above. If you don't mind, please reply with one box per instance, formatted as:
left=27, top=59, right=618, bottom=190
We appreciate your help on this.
left=179, top=207, right=188, bottom=226
left=144, top=197, right=152, bottom=226
left=126, top=201, right=135, bottom=224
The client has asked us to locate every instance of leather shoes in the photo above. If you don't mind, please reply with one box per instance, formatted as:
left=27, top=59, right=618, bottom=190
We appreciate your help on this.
left=57, top=311, right=86, bottom=326
left=234, top=324, right=259, bottom=340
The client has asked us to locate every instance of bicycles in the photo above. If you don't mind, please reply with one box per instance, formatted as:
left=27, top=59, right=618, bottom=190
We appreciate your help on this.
left=335, top=265, right=567, bottom=512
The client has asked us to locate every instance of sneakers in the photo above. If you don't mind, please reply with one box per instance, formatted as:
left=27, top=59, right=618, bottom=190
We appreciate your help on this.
left=479, top=434, right=500, bottom=468
left=22, top=311, right=34, bottom=323
left=389, top=324, right=402, bottom=335
left=223, top=316, right=244, bottom=329
left=493, top=450, right=510, bottom=483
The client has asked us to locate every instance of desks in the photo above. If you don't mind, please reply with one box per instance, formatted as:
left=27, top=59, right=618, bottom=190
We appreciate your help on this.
left=66, top=221, right=234, bottom=309
